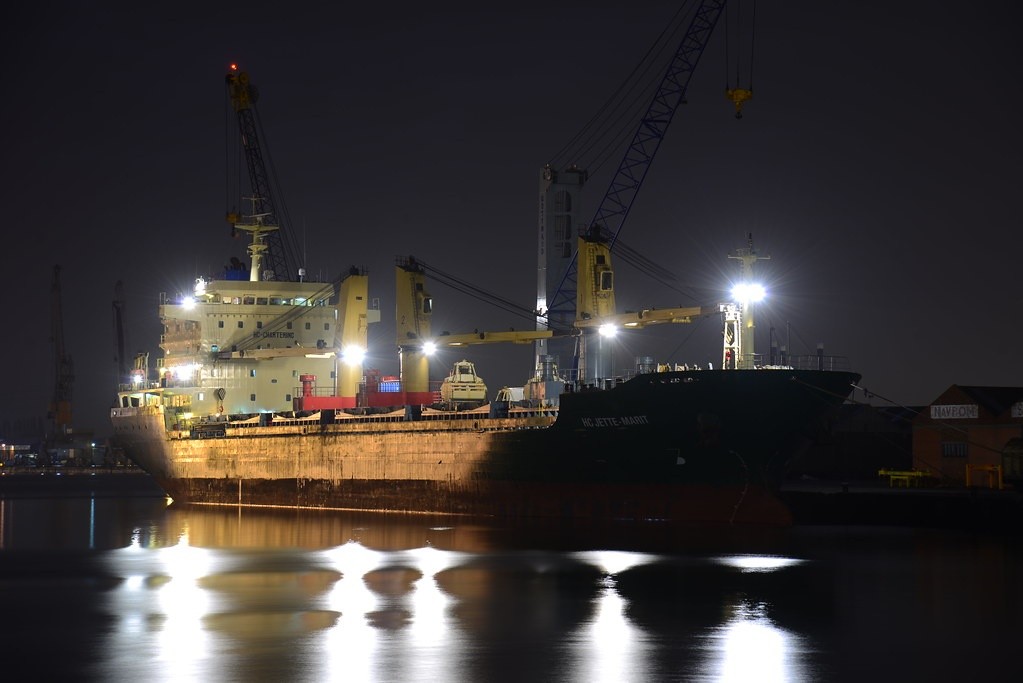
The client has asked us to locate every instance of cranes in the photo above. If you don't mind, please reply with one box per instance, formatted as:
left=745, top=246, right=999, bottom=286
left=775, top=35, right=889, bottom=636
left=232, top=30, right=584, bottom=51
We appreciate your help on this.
left=111, top=278, right=133, bottom=388
left=44, top=262, right=81, bottom=431
left=525, top=1, right=765, bottom=394
left=211, top=62, right=305, bottom=280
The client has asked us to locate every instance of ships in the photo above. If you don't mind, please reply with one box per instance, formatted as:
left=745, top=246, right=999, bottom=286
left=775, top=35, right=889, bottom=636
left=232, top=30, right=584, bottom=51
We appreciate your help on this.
left=107, top=272, right=870, bottom=544
